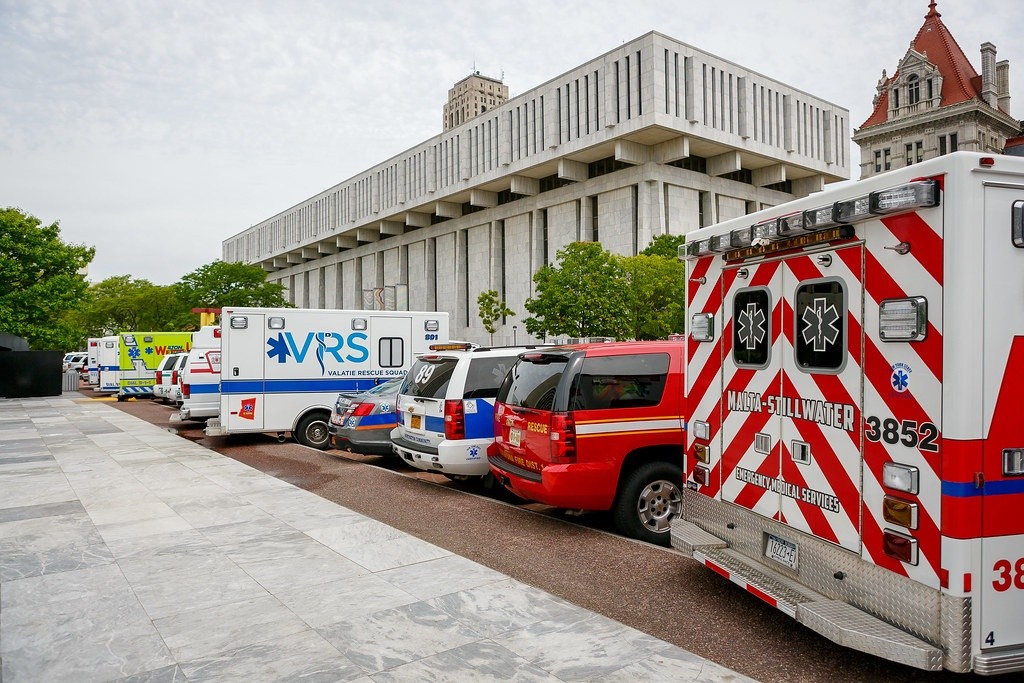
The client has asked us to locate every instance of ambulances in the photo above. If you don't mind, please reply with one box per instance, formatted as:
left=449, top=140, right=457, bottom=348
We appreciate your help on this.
left=203, top=307, right=448, bottom=440
left=168, top=326, right=222, bottom=425
left=672, top=152, right=1023, bottom=677
left=119, top=331, right=195, bottom=404
left=87, top=336, right=120, bottom=393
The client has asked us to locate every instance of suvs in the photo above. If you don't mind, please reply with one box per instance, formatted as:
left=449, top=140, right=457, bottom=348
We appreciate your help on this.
left=68, top=355, right=88, bottom=381
left=63, top=352, right=88, bottom=374
left=487, top=335, right=683, bottom=548
left=389, top=341, right=566, bottom=481
left=154, top=353, right=190, bottom=408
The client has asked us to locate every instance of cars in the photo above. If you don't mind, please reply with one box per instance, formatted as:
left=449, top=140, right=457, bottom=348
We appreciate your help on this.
left=327, top=377, right=404, bottom=456
